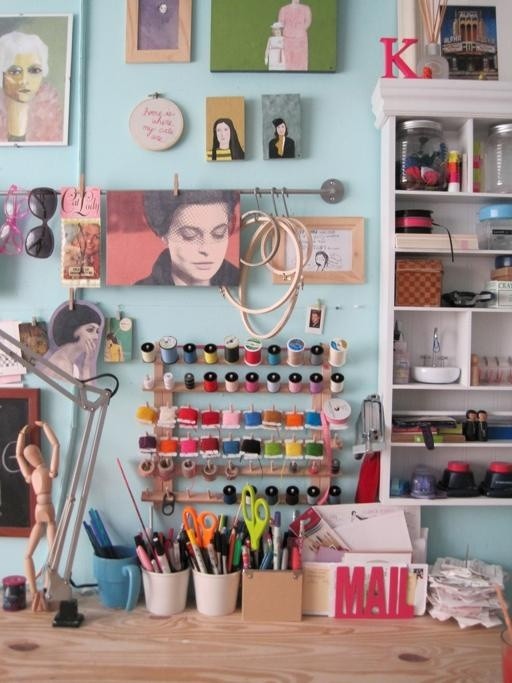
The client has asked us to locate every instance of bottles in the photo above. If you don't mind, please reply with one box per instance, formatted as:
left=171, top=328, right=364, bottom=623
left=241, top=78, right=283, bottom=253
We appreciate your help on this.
left=481, top=122, right=512, bottom=194
left=394, top=118, right=450, bottom=192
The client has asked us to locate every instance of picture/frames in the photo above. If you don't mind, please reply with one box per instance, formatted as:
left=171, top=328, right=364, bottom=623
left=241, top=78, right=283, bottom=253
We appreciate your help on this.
left=396, top=0, right=509, bottom=81
left=123, top=0, right=192, bottom=64
left=273, top=217, right=366, bottom=286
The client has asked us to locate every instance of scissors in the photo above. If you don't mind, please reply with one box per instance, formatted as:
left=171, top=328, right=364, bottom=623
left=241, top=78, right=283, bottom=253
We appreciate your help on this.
left=241, top=485, right=270, bottom=570
left=182, top=506, right=220, bottom=574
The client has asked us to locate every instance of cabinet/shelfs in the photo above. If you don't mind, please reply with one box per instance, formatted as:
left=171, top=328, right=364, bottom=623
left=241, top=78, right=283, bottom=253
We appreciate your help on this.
left=371, top=77, right=512, bottom=506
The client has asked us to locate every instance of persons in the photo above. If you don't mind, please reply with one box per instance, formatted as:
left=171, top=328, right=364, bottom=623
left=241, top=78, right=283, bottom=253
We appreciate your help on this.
left=41, top=303, right=102, bottom=385
left=277, top=0, right=313, bottom=70
left=146, top=1, right=178, bottom=50
left=208, top=117, right=245, bottom=161
left=65, top=220, right=100, bottom=278
left=105, top=331, right=126, bottom=361
left=267, top=116, right=295, bottom=157
left=263, top=19, right=284, bottom=72
left=14, top=419, right=63, bottom=611
left=310, top=310, right=319, bottom=327
left=132, top=189, right=239, bottom=285
left=0, top=27, right=63, bottom=143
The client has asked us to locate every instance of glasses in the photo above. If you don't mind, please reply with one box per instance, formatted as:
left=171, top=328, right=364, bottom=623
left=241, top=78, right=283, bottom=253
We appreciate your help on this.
left=26, top=189, right=58, bottom=259
left=0, top=186, right=29, bottom=256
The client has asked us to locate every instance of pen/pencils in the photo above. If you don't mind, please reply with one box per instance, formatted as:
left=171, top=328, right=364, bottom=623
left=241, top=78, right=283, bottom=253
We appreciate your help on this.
left=241, top=509, right=305, bottom=570
left=133, top=523, right=189, bottom=574
left=82, top=507, right=118, bottom=559
left=180, top=513, right=245, bottom=574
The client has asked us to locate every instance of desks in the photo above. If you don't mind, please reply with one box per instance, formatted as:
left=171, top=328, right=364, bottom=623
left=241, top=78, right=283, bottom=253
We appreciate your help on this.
left=0, top=586, right=512, bottom=683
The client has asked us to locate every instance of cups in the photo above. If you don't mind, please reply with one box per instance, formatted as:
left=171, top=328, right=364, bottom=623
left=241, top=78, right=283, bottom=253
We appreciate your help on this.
left=91, top=543, right=141, bottom=614
left=192, top=567, right=241, bottom=615
left=138, top=567, right=190, bottom=619
